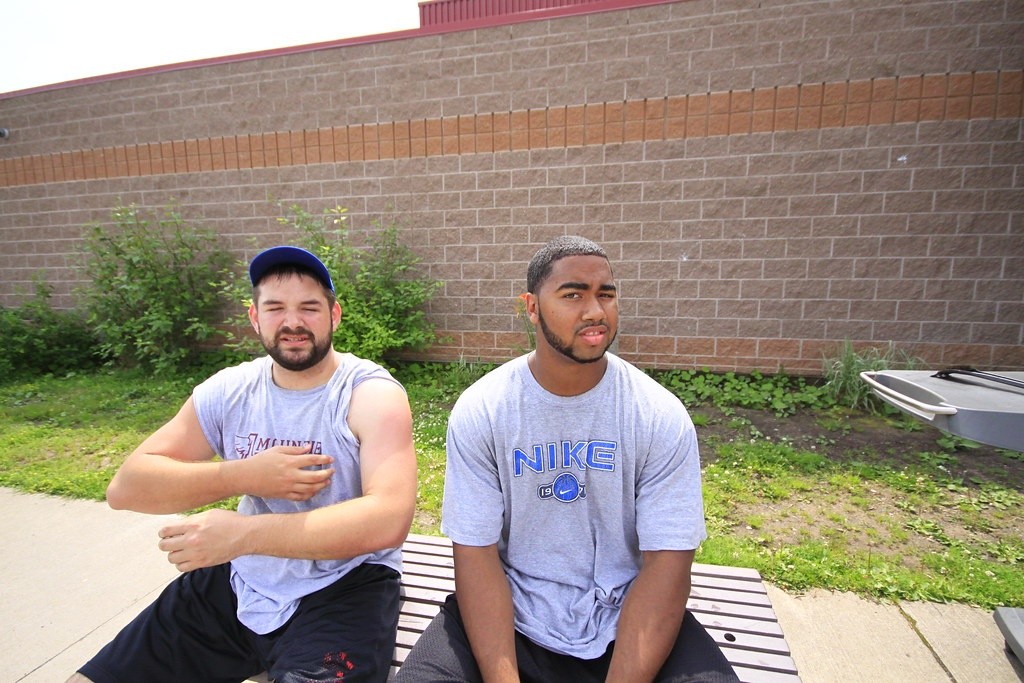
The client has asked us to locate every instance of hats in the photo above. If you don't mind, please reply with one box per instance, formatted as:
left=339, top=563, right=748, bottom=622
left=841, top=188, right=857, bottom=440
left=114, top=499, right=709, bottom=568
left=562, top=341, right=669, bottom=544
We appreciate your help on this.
left=249, top=245, right=335, bottom=294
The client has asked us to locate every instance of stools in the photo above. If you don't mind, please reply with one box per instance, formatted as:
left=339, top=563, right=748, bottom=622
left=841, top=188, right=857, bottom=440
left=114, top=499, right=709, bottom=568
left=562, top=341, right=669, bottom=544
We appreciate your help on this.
left=243, top=531, right=803, bottom=683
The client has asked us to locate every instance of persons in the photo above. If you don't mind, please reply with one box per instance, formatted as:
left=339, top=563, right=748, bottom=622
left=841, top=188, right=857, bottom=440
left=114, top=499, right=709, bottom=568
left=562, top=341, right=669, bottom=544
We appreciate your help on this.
left=390, top=235, right=744, bottom=681
left=63, top=245, right=417, bottom=683
